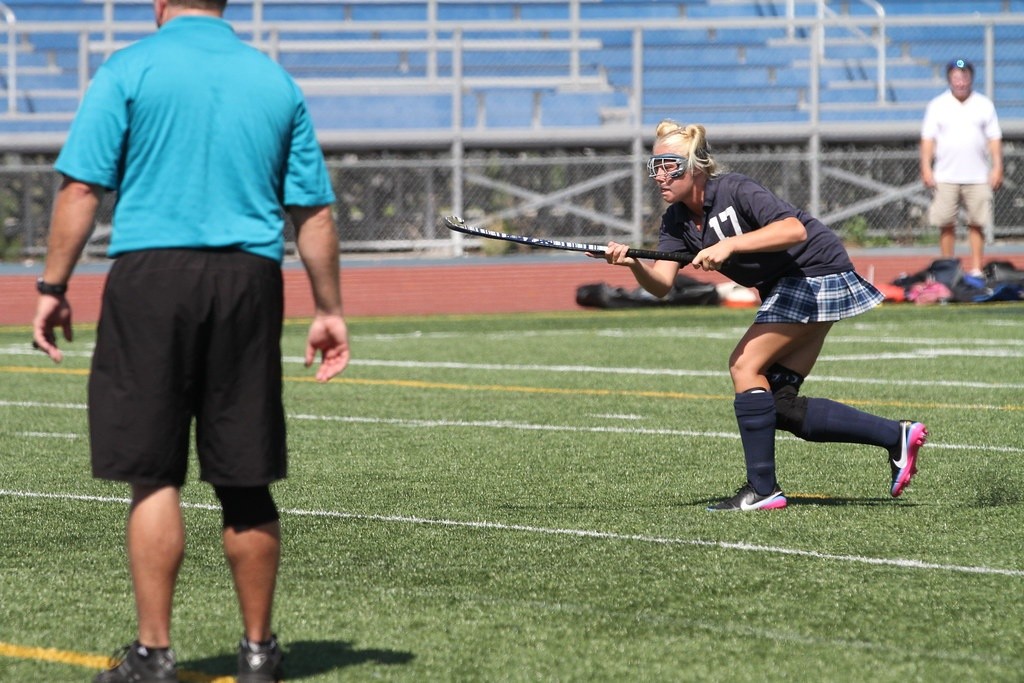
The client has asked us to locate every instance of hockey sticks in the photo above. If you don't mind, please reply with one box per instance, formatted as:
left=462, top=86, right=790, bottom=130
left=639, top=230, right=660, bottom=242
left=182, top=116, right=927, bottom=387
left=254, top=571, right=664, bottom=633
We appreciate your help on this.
left=444, top=215, right=708, bottom=264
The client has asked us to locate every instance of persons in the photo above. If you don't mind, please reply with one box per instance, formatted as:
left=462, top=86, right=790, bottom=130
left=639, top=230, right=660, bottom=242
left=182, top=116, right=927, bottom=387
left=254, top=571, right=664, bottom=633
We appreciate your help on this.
left=584, top=118, right=929, bottom=512
left=30, top=0, right=352, bottom=683
left=919, top=58, right=1005, bottom=279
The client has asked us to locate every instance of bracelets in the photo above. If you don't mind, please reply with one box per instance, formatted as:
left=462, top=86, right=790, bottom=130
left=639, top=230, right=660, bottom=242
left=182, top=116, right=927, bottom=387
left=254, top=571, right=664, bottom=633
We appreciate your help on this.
left=36, top=277, right=68, bottom=294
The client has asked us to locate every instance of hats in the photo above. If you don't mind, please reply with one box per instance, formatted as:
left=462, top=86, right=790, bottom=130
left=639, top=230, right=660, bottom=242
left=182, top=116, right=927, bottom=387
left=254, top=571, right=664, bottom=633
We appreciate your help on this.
left=945, top=57, right=975, bottom=80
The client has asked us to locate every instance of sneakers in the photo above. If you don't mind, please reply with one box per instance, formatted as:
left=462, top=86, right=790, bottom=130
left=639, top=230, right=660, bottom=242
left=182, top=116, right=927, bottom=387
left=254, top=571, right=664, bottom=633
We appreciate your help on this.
left=235, top=634, right=283, bottom=683
left=887, top=419, right=929, bottom=497
left=93, top=640, right=177, bottom=683
left=706, top=482, right=787, bottom=512
